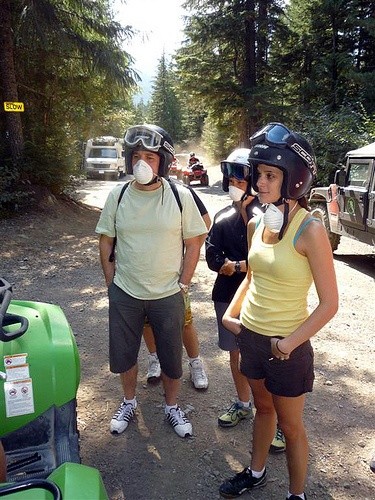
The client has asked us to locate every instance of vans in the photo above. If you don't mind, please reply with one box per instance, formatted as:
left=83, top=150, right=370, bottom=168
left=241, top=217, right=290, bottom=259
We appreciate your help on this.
left=84, top=135, right=127, bottom=181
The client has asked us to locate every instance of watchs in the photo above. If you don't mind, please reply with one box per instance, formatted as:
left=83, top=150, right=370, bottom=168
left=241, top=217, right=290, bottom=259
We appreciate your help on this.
left=178, top=282, right=188, bottom=293
left=235, top=261, right=241, bottom=271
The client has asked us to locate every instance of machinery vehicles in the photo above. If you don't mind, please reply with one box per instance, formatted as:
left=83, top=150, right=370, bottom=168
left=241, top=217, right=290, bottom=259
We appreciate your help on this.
left=1, top=277, right=111, bottom=499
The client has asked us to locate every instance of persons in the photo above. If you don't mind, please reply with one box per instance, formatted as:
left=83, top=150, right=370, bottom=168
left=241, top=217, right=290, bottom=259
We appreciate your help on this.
left=95, top=124, right=209, bottom=438
left=219, top=122, right=338, bottom=500
left=205, top=148, right=285, bottom=452
left=189, top=152, right=199, bottom=166
left=142, top=155, right=211, bottom=389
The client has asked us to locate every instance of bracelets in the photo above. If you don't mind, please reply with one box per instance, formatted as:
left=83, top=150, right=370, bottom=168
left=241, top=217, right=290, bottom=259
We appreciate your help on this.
left=276, top=340, right=288, bottom=355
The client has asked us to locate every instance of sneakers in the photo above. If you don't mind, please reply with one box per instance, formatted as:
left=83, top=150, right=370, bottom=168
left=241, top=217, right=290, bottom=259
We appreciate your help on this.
left=286, top=492, right=307, bottom=500
left=147, top=354, right=162, bottom=382
left=110, top=395, right=137, bottom=434
left=219, top=465, right=267, bottom=497
left=188, top=358, right=208, bottom=389
left=218, top=401, right=254, bottom=427
left=164, top=403, right=193, bottom=437
left=270, top=423, right=287, bottom=452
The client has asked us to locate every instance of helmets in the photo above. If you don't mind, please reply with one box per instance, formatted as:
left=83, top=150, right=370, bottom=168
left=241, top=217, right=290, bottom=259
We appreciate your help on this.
left=247, top=132, right=316, bottom=199
left=222, top=147, right=258, bottom=196
left=122, top=123, right=177, bottom=176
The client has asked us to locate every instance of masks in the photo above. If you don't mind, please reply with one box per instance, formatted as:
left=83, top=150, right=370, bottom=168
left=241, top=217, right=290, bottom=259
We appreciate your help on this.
left=262, top=201, right=298, bottom=234
left=228, top=186, right=245, bottom=202
left=132, top=159, right=158, bottom=184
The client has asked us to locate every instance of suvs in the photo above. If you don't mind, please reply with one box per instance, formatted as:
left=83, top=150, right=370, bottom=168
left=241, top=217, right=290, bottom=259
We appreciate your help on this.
left=308, top=141, right=375, bottom=253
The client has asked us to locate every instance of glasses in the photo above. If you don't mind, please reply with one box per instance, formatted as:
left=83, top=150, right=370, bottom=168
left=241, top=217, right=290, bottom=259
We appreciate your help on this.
left=249, top=122, right=292, bottom=149
left=221, top=160, right=251, bottom=181
left=125, top=125, right=163, bottom=152
